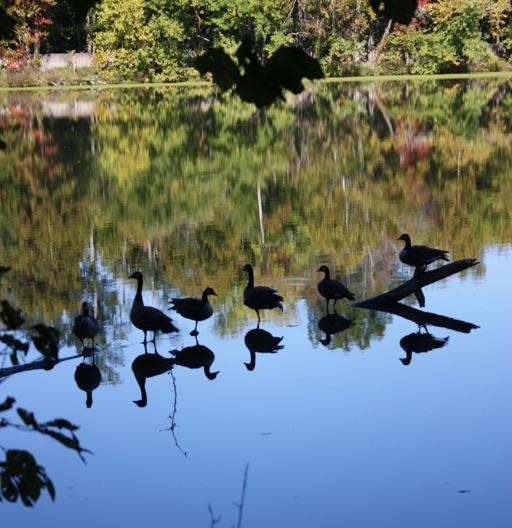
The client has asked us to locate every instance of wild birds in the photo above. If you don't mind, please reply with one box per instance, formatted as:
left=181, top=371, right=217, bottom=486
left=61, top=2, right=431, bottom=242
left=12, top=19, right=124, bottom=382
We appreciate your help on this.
left=127, top=270, right=179, bottom=344
left=131, top=344, right=175, bottom=409
left=317, top=310, right=357, bottom=347
left=73, top=301, right=99, bottom=350
left=243, top=323, right=286, bottom=371
left=167, top=286, right=218, bottom=332
left=242, top=263, right=283, bottom=321
left=396, top=232, right=449, bottom=274
left=173, top=335, right=220, bottom=381
left=398, top=325, right=449, bottom=367
left=315, top=265, right=356, bottom=309
left=74, top=355, right=102, bottom=409
